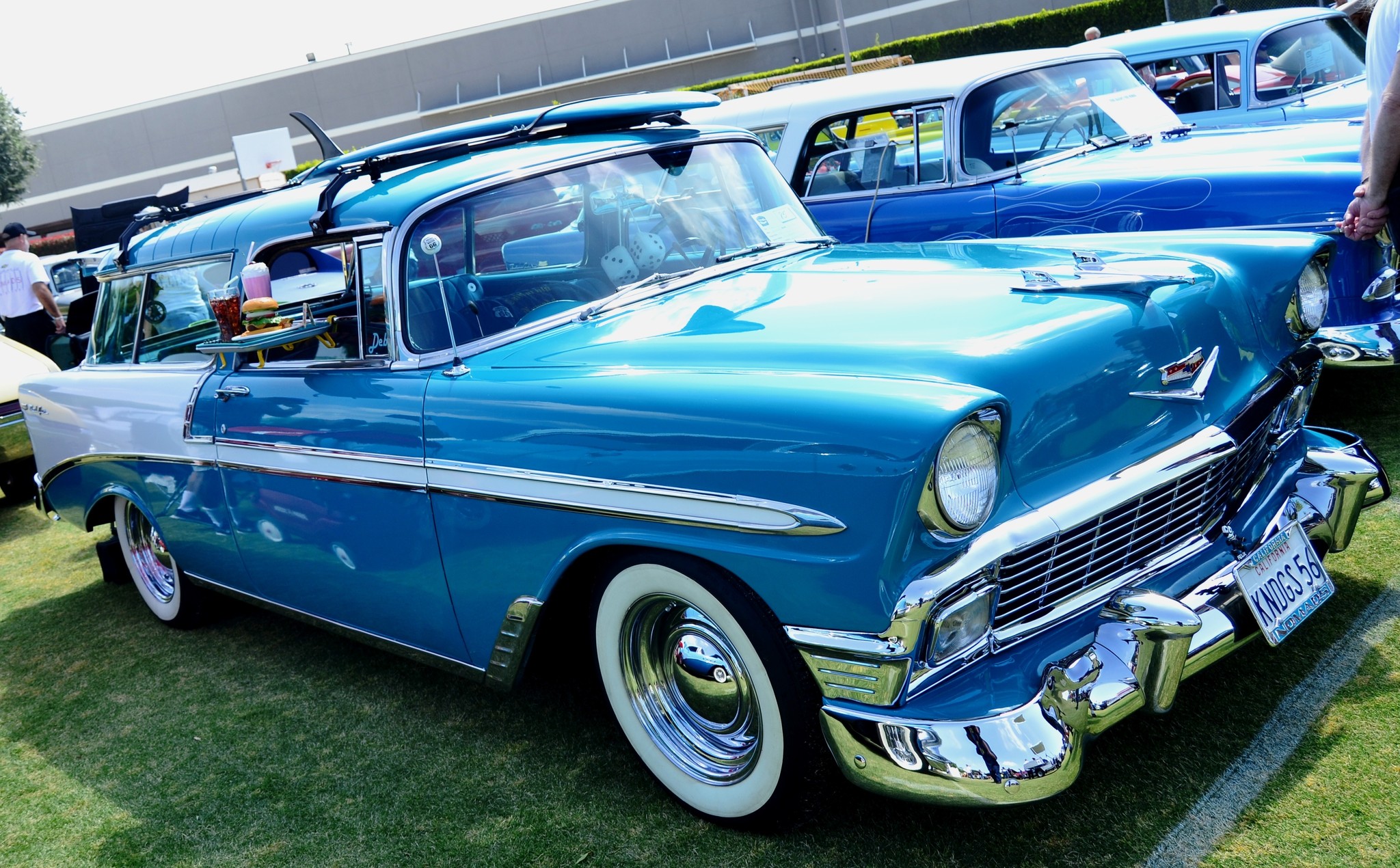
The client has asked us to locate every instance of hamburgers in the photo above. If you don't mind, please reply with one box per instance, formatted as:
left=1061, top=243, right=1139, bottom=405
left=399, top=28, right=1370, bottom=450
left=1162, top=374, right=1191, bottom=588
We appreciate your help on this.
left=241, top=298, right=282, bottom=335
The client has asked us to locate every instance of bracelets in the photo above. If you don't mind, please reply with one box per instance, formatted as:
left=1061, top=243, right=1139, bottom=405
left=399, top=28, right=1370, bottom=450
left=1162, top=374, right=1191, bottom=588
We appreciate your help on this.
left=1361, top=175, right=1368, bottom=184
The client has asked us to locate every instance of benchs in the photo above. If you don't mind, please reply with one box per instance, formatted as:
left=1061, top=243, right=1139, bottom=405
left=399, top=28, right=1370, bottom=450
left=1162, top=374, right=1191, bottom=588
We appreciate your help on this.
left=158, top=273, right=484, bottom=366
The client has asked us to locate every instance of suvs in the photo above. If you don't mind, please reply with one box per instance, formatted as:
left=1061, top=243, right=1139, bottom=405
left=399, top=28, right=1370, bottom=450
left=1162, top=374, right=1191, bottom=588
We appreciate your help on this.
left=15, top=88, right=1394, bottom=825
left=685, top=46, right=1400, bottom=416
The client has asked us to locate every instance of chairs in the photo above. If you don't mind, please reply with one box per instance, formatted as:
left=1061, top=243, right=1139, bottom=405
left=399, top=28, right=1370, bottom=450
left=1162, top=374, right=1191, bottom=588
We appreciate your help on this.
left=802, top=165, right=917, bottom=194
left=1175, top=84, right=1233, bottom=114
left=409, top=309, right=474, bottom=356
left=909, top=156, right=995, bottom=182
left=464, top=276, right=612, bottom=337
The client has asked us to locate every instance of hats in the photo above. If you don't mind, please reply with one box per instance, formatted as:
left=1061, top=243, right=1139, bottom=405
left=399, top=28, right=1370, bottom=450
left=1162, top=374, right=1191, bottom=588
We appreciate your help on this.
left=2, top=222, right=36, bottom=241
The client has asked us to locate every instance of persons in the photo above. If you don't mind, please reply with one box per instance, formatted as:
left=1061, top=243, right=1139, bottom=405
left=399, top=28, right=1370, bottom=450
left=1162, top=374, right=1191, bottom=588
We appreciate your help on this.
left=1335, top=0, right=1399, bottom=318
left=130, top=205, right=210, bottom=340
left=807, top=157, right=840, bottom=176
left=0, top=223, right=66, bottom=361
left=1132, top=64, right=1157, bottom=92
left=1084, top=27, right=1100, bottom=41
left=1208, top=4, right=1242, bottom=66
left=164, top=461, right=257, bottom=539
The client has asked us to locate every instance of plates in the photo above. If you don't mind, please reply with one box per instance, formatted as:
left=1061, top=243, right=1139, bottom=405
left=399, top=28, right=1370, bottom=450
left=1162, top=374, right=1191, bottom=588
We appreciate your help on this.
left=231, top=323, right=304, bottom=342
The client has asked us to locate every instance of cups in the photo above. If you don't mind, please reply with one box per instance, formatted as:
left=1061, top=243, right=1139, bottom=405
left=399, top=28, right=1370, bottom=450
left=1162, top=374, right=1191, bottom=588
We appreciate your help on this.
left=240, top=267, right=272, bottom=300
left=207, top=287, right=244, bottom=342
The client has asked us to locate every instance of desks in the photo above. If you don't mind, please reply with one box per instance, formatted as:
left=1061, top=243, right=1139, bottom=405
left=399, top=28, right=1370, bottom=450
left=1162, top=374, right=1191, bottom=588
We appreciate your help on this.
left=195, top=321, right=336, bottom=370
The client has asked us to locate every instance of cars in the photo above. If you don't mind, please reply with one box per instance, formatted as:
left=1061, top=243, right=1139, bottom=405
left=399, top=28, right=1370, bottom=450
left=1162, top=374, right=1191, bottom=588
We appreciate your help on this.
left=0, top=321, right=62, bottom=503
left=38, top=243, right=113, bottom=323
left=1055, top=7, right=1370, bottom=129
